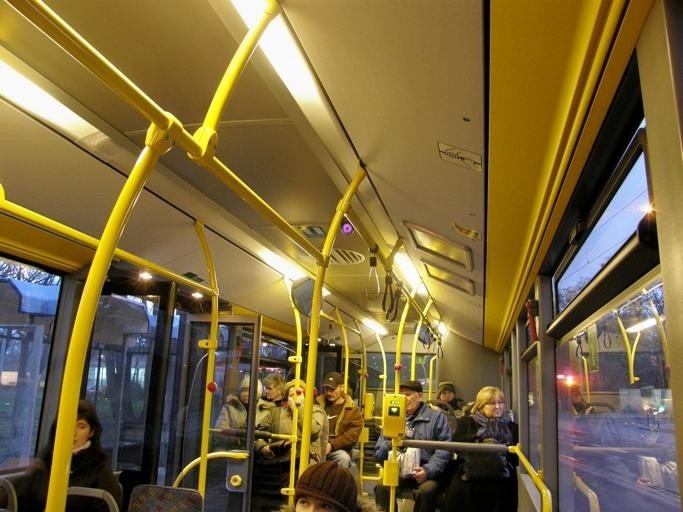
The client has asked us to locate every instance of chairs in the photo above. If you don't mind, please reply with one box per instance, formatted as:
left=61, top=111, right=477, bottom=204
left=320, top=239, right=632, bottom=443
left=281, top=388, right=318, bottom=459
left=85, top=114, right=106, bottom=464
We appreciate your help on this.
left=0, top=399, right=465, bottom=512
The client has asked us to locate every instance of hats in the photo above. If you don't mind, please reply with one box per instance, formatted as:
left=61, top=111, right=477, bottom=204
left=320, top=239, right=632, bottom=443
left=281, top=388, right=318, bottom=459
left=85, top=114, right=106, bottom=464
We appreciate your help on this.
left=400, top=381, right=423, bottom=392
left=321, top=372, right=344, bottom=389
left=239, top=376, right=263, bottom=394
left=283, top=378, right=306, bottom=398
left=438, top=382, right=455, bottom=395
left=296, top=461, right=357, bottom=511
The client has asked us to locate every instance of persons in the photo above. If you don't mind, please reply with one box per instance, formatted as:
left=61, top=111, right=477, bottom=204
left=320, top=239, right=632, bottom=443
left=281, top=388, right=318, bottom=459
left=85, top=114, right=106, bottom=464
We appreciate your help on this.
left=193, top=371, right=383, bottom=511
left=5, top=399, right=121, bottom=512
left=374, top=381, right=452, bottom=512
left=435, top=381, right=466, bottom=414
left=441, top=385, right=519, bottom=512
left=571, top=372, right=680, bottom=511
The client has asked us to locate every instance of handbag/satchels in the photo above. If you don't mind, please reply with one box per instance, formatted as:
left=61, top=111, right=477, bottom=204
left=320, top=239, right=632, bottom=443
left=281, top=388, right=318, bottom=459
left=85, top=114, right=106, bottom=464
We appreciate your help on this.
left=462, top=436, right=497, bottom=467
